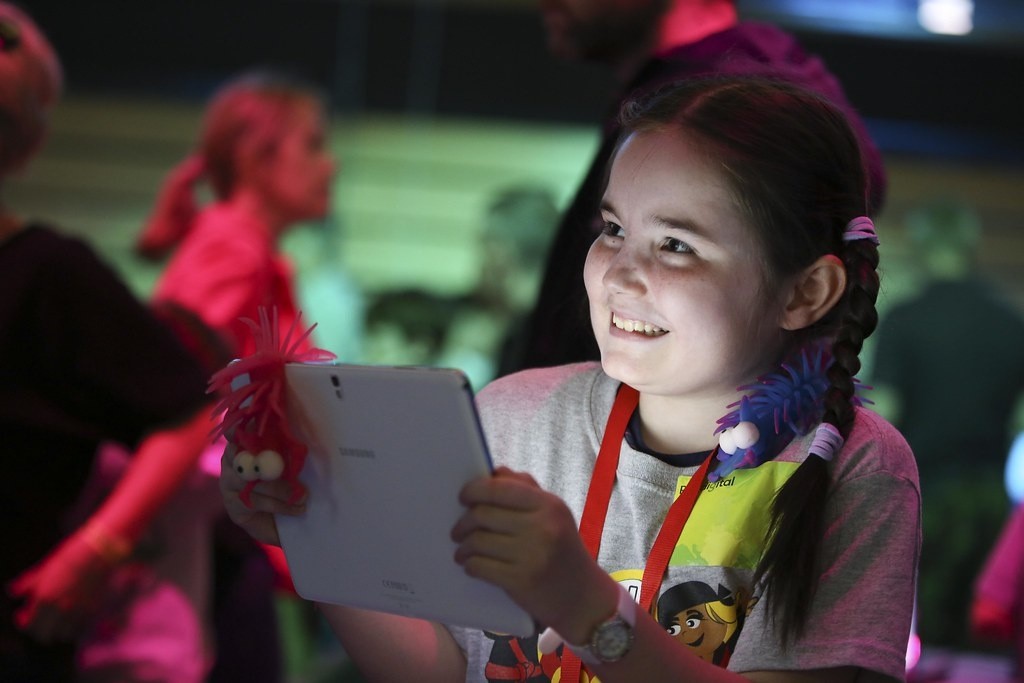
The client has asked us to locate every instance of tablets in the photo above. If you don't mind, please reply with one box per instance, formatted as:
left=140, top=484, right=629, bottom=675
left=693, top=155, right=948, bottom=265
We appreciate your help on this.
left=226, top=359, right=541, bottom=640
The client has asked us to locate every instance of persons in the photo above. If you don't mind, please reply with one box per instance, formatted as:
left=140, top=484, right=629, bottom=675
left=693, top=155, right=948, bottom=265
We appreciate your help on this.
left=853, top=203, right=1024, bottom=683
left=0, top=0, right=336, bottom=683
left=220, top=74, right=920, bottom=683
left=494, top=0, right=886, bottom=381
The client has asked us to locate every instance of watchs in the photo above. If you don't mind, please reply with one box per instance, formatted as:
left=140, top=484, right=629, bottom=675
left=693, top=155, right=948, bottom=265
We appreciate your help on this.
left=564, top=582, right=635, bottom=664
